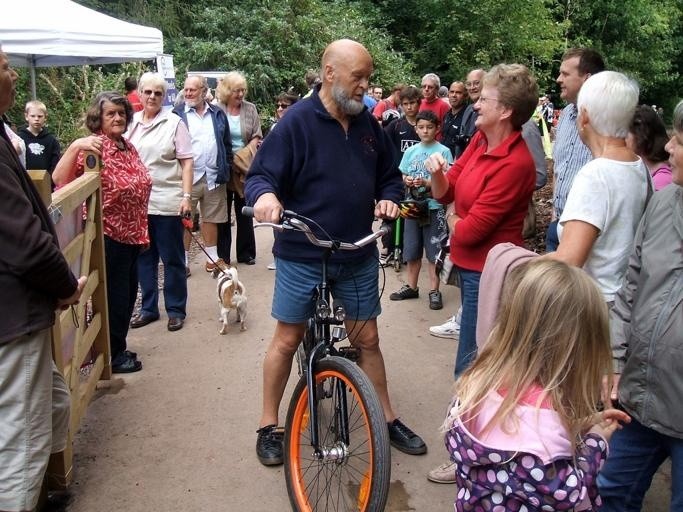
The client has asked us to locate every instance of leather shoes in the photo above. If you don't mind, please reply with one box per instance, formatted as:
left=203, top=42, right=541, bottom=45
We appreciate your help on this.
left=131, top=313, right=160, bottom=327
left=167, top=313, right=184, bottom=331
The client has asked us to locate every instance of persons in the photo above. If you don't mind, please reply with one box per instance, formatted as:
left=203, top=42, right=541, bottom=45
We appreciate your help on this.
left=372, top=84, right=404, bottom=121
left=455, top=67, right=487, bottom=160
left=439, top=81, right=468, bottom=156
left=389, top=108, right=454, bottom=311
left=0, top=43, right=91, bottom=511
left=242, top=36, right=431, bottom=468
left=170, top=74, right=234, bottom=279
left=436, top=252, right=629, bottom=512
left=50, top=90, right=153, bottom=373
left=215, top=71, right=262, bottom=265
left=438, top=85, right=450, bottom=107
left=531, top=92, right=555, bottom=138
left=418, top=73, right=451, bottom=141
left=366, top=84, right=383, bottom=113
left=128, top=73, right=195, bottom=332
left=427, top=116, right=548, bottom=340
left=424, top=60, right=539, bottom=487
left=486, top=68, right=652, bottom=309
left=121, top=75, right=145, bottom=113
left=382, top=85, right=423, bottom=158
left=626, top=104, right=674, bottom=193
left=276, top=91, right=300, bottom=119
left=543, top=47, right=608, bottom=254
left=591, top=101, right=683, bottom=510
left=17, top=98, right=64, bottom=192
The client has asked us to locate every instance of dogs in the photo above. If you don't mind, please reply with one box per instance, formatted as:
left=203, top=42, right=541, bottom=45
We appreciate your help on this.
left=211, top=265, right=248, bottom=334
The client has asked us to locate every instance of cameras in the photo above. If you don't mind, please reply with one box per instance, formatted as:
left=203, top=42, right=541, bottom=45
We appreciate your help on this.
left=452, top=134, right=470, bottom=151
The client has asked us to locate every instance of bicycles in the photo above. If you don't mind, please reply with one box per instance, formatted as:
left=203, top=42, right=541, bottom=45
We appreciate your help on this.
left=241, top=207, right=399, bottom=512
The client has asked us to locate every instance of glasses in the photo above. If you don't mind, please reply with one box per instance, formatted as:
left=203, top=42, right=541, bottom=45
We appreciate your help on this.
left=465, top=80, right=480, bottom=85
left=141, top=89, right=163, bottom=97
left=274, top=103, right=291, bottom=109
left=478, top=95, right=498, bottom=103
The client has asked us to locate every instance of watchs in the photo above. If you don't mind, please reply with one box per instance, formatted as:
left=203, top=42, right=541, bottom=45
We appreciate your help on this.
left=183, top=192, right=192, bottom=200
left=445, top=209, right=459, bottom=222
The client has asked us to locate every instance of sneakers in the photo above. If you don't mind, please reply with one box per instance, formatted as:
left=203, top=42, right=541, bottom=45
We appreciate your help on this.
left=206, top=258, right=237, bottom=272
left=429, top=288, right=443, bottom=310
left=389, top=284, right=420, bottom=300
left=428, top=315, right=462, bottom=341
left=254, top=422, right=284, bottom=465
left=268, top=262, right=276, bottom=270
left=382, top=418, right=427, bottom=456
left=426, top=463, right=461, bottom=484
left=380, top=249, right=396, bottom=268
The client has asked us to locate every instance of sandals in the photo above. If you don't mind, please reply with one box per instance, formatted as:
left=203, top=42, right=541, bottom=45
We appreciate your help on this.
left=123, top=350, right=138, bottom=360
left=111, top=359, right=142, bottom=373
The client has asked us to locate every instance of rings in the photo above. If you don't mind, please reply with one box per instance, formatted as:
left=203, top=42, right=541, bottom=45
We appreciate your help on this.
left=428, top=166, right=435, bottom=172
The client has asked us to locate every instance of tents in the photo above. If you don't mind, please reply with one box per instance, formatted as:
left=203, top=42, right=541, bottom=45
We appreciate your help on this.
left=1, top=0, right=166, bottom=62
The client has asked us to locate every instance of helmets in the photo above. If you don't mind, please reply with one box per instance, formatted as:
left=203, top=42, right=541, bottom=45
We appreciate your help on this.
left=398, top=200, right=429, bottom=220
